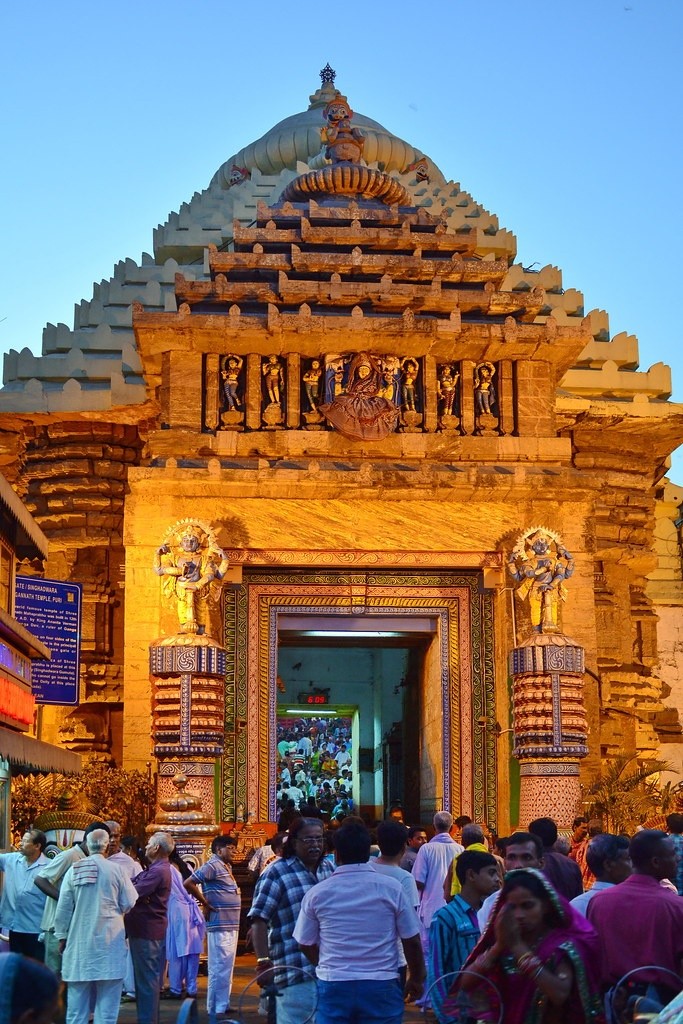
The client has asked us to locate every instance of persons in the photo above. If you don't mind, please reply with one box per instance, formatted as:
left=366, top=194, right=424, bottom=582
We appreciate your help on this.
left=411, top=811, right=465, bottom=1009
left=302, top=357, right=497, bottom=415
left=424, top=851, right=500, bottom=1024
left=666, top=813, right=683, bottom=897
left=569, top=834, right=633, bottom=917
left=509, top=537, right=574, bottom=630
left=401, top=826, right=427, bottom=873
left=528, top=818, right=583, bottom=902
left=0, top=714, right=428, bottom=1024
left=461, top=868, right=601, bottom=1024
left=443, top=816, right=503, bottom=903
left=586, top=830, right=683, bottom=1024
left=569, top=817, right=588, bottom=860
left=576, top=819, right=604, bottom=892
left=477, top=831, right=545, bottom=936
left=156, top=530, right=229, bottom=634
left=221, top=354, right=244, bottom=413
left=262, top=355, right=285, bottom=405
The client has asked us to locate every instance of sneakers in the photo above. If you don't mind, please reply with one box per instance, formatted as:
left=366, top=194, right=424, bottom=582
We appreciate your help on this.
left=160, top=990, right=181, bottom=999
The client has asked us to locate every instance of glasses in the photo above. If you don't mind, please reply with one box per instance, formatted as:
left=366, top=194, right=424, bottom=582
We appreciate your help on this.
left=227, top=846, right=238, bottom=849
left=297, top=837, right=327, bottom=843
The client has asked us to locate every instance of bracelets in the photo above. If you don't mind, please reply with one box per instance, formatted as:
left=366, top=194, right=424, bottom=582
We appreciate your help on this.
left=516, top=951, right=545, bottom=983
left=257, top=957, right=271, bottom=962
